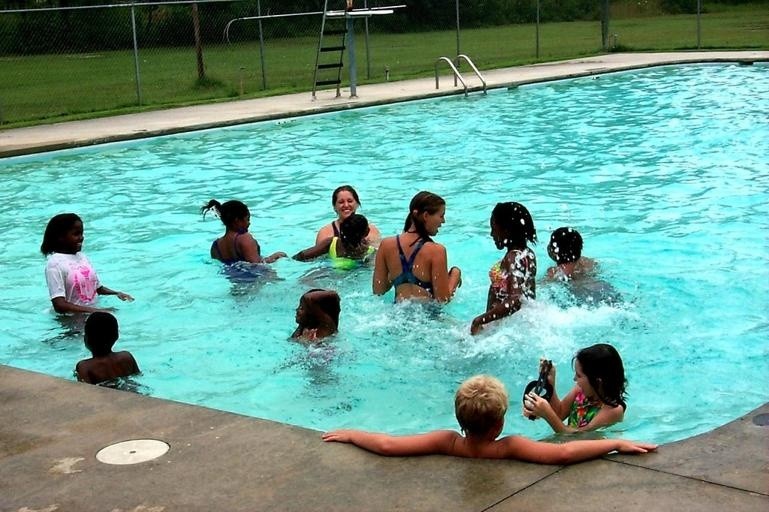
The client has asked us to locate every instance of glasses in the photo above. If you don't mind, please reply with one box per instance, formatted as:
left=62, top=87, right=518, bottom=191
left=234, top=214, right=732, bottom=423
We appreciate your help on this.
left=522, top=359, right=556, bottom=421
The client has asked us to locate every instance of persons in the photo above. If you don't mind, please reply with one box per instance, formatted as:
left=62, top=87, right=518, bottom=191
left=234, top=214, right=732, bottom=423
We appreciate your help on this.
left=288, top=289, right=340, bottom=349
left=545, top=226, right=596, bottom=282
left=291, top=214, right=377, bottom=274
left=201, top=199, right=287, bottom=283
left=523, top=343, right=627, bottom=443
left=316, top=185, right=381, bottom=251
left=320, top=374, right=661, bottom=466
left=39, top=213, right=135, bottom=316
left=371, top=191, right=461, bottom=316
left=76, top=311, right=140, bottom=385
left=470, top=202, right=536, bottom=336
